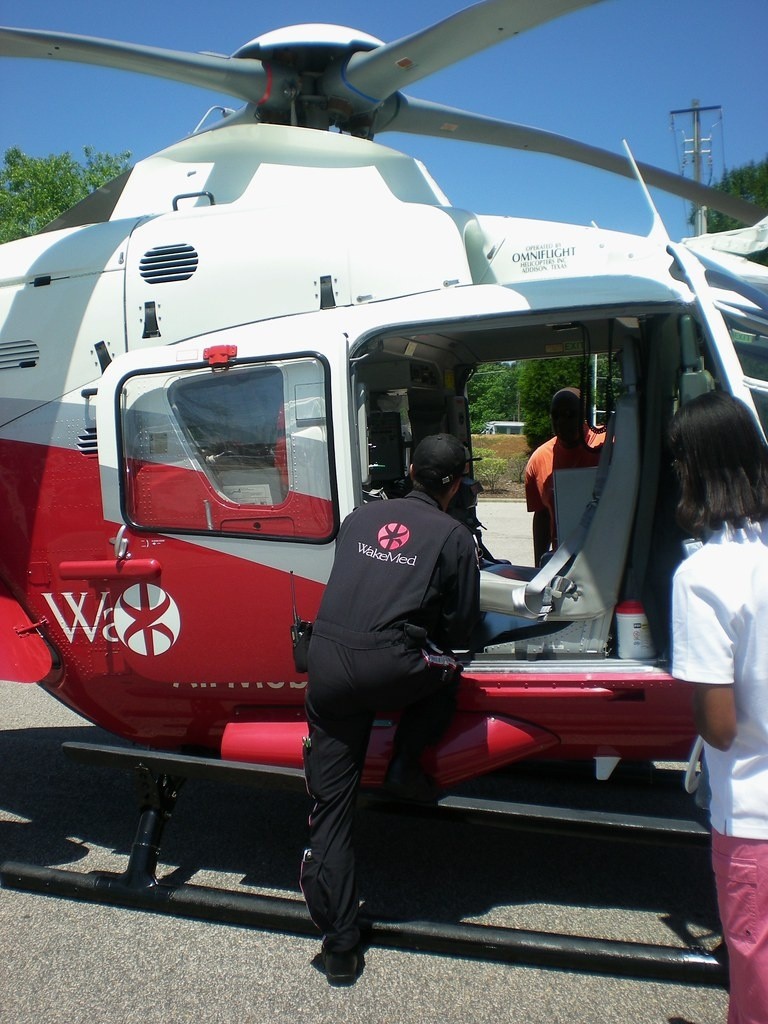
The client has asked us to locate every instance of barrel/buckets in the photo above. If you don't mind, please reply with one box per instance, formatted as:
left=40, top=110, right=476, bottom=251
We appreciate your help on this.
left=616, top=600, right=657, bottom=659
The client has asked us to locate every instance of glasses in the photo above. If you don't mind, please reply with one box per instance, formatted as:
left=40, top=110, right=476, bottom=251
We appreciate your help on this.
left=552, top=409, right=580, bottom=419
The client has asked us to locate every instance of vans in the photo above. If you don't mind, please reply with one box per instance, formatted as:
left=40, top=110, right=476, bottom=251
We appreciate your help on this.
left=482, top=420, right=528, bottom=436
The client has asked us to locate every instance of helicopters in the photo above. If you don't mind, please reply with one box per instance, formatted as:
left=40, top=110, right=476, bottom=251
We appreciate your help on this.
left=0, top=0, right=766, bottom=995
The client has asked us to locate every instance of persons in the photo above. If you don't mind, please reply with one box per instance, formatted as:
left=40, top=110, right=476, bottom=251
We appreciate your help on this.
left=671, top=390, right=768, bottom=1024
left=525, top=386, right=616, bottom=568
left=299, top=432, right=481, bottom=986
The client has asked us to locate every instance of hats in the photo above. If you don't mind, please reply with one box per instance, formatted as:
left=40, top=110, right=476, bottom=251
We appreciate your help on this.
left=410, top=431, right=467, bottom=485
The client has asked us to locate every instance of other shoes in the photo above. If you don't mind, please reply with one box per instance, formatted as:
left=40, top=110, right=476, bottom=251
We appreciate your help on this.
left=321, top=944, right=360, bottom=984
left=380, top=776, right=447, bottom=801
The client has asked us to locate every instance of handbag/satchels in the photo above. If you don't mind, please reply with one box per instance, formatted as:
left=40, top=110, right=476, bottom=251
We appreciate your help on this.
left=684, top=735, right=711, bottom=810
